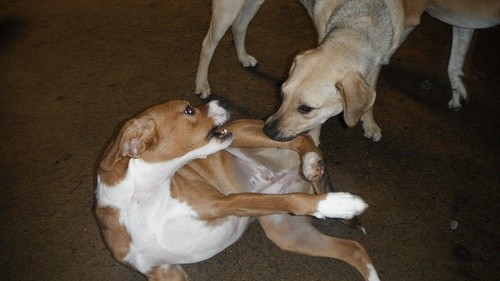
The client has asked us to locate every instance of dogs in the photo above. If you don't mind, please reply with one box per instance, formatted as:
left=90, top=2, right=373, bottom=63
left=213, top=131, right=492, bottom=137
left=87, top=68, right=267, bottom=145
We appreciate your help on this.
left=194, top=0, right=500, bottom=143
left=94, top=98, right=380, bottom=280
